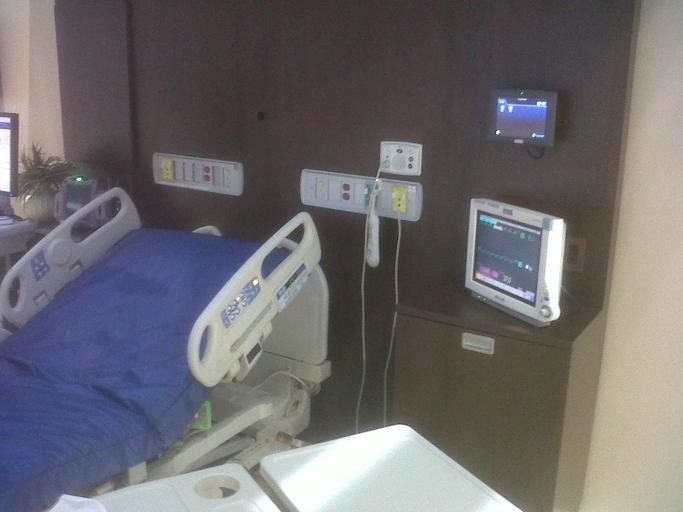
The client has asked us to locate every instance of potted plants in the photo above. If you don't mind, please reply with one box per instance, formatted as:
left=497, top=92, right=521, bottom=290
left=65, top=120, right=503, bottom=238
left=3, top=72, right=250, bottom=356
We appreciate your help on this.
left=16, top=141, right=71, bottom=223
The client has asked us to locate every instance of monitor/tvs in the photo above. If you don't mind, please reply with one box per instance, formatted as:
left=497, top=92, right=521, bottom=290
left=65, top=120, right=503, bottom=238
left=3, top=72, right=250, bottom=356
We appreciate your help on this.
left=58, top=176, right=97, bottom=225
left=487, top=87, right=563, bottom=150
left=464, top=196, right=567, bottom=328
left=0, top=112, right=19, bottom=198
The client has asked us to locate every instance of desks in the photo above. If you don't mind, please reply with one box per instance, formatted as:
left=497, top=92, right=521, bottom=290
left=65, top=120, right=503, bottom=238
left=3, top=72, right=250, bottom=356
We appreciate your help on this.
left=0, top=185, right=331, bottom=512
left=0, top=216, right=90, bottom=274
left=91, top=423, right=521, bottom=512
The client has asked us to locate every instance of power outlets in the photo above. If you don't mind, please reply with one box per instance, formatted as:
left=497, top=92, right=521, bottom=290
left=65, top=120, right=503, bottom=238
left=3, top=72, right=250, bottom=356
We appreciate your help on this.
left=380, top=142, right=423, bottom=176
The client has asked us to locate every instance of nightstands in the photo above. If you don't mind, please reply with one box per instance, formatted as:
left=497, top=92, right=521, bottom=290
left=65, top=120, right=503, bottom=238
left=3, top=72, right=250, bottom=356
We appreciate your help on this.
left=393, top=285, right=604, bottom=512
